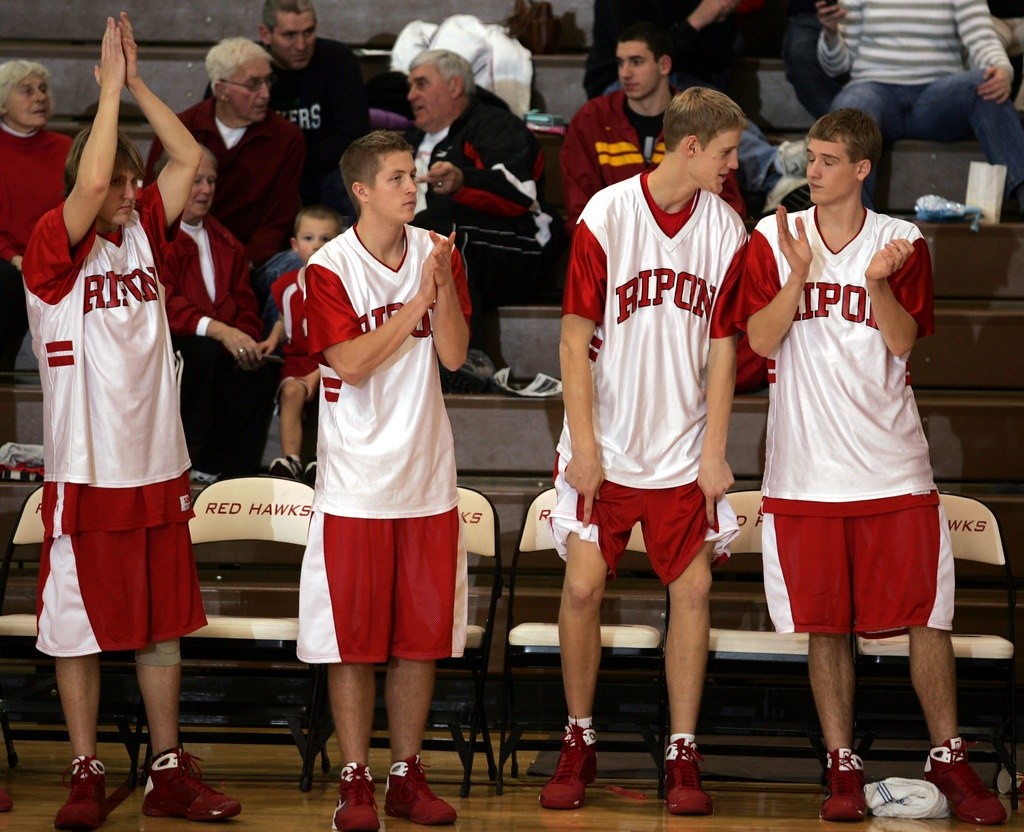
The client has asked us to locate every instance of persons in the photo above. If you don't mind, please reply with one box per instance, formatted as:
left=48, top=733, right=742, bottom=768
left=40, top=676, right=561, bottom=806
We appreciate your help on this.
left=21, top=11, right=243, bottom=830
left=535, top=86, right=751, bottom=816
left=396, top=50, right=545, bottom=393
left=581, top=0, right=852, bottom=216
left=305, top=130, right=472, bottom=831
left=255, top=204, right=347, bottom=489
left=815, top=0, right=1024, bottom=217
left=139, top=34, right=307, bottom=281
left=153, top=144, right=267, bottom=486
left=202, top=0, right=536, bottom=227
left=557, top=25, right=746, bottom=238
left=745, top=108, right=1010, bottom=826
left=0, top=60, right=77, bottom=387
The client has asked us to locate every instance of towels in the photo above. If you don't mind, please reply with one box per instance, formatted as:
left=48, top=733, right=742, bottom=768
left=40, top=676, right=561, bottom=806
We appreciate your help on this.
left=0, top=442, right=44, bottom=468
left=863, top=777, right=951, bottom=820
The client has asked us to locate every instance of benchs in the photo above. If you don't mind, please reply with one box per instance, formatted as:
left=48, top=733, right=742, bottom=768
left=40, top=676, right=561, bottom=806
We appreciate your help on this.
left=0, top=0, right=1024, bottom=672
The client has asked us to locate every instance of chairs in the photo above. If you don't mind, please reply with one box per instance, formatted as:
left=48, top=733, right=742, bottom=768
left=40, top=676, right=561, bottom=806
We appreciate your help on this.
left=495, top=485, right=673, bottom=800
left=138, top=472, right=329, bottom=791
left=850, top=490, right=1021, bottom=817
left=299, top=487, right=507, bottom=799
left=0, top=468, right=145, bottom=812
left=690, top=487, right=838, bottom=802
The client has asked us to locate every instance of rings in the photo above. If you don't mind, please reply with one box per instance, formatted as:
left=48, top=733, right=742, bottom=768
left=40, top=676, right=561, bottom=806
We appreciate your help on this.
left=437, top=182, right=443, bottom=187
left=238, top=349, right=244, bottom=353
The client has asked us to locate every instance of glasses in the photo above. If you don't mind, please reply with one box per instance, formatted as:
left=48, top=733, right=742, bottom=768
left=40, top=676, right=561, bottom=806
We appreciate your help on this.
left=219, top=79, right=273, bottom=91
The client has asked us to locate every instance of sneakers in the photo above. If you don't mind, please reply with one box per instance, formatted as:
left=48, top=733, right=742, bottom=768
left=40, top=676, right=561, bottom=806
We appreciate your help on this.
left=922, top=736, right=1007, bottom=824
left=539, top=715, right=597, bottom=808
left=820, top=747, right=867, bottom=819
left=54, top=755, right=107, bottom=829
left=332, top=762, right=380, bottom=832
left=141, top=741, right=240, bottom=819
left=384, top=753, right=457, bottom=824
left=664, top=738, right=711, bottom=813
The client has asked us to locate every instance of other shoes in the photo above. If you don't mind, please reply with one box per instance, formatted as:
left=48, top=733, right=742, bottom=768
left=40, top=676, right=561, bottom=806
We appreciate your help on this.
left=762, top=176, right=810, bottom=216
left=269, top=455, right=302, bottom=479
left=305, top=462, right=317, bottom=484
left=774, top=140, right=809, bottom=175
